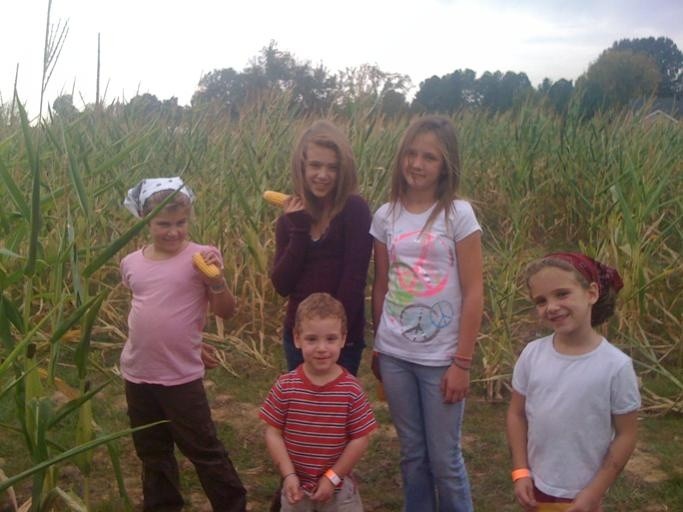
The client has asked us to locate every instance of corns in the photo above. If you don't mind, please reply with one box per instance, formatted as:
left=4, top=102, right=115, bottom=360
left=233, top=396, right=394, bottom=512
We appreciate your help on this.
left=262, top=191, right=288, bottom=208
left=75, top=387, right=94, bottom=477
left=193, top=251, right=221, bottom=282
left=23, top=345, right=44, bottom=448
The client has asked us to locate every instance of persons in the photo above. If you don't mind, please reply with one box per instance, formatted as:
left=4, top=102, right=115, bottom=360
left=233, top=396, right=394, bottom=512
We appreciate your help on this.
left=507, top=251, right=643, bottom=512
left=270, top=119, right=373, bottom=512
left=366, top=115, right=485, bottom=512
left=258, top=292, right=379, bottom=512
left=118, top=176, right=248, bottom=512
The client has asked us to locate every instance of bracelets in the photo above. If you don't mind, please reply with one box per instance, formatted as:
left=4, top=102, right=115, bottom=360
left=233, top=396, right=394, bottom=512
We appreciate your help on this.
left=453, top=354, right=473, bottom=369
left=511, top=468, right=532, bottom=483
left=371, top=350, right=379, bottom=358
left=324, top=468, right=343, bottom=487
left=208, top=277, right=226, bottom=294
left=282, top=472, right=297, bottom=480
